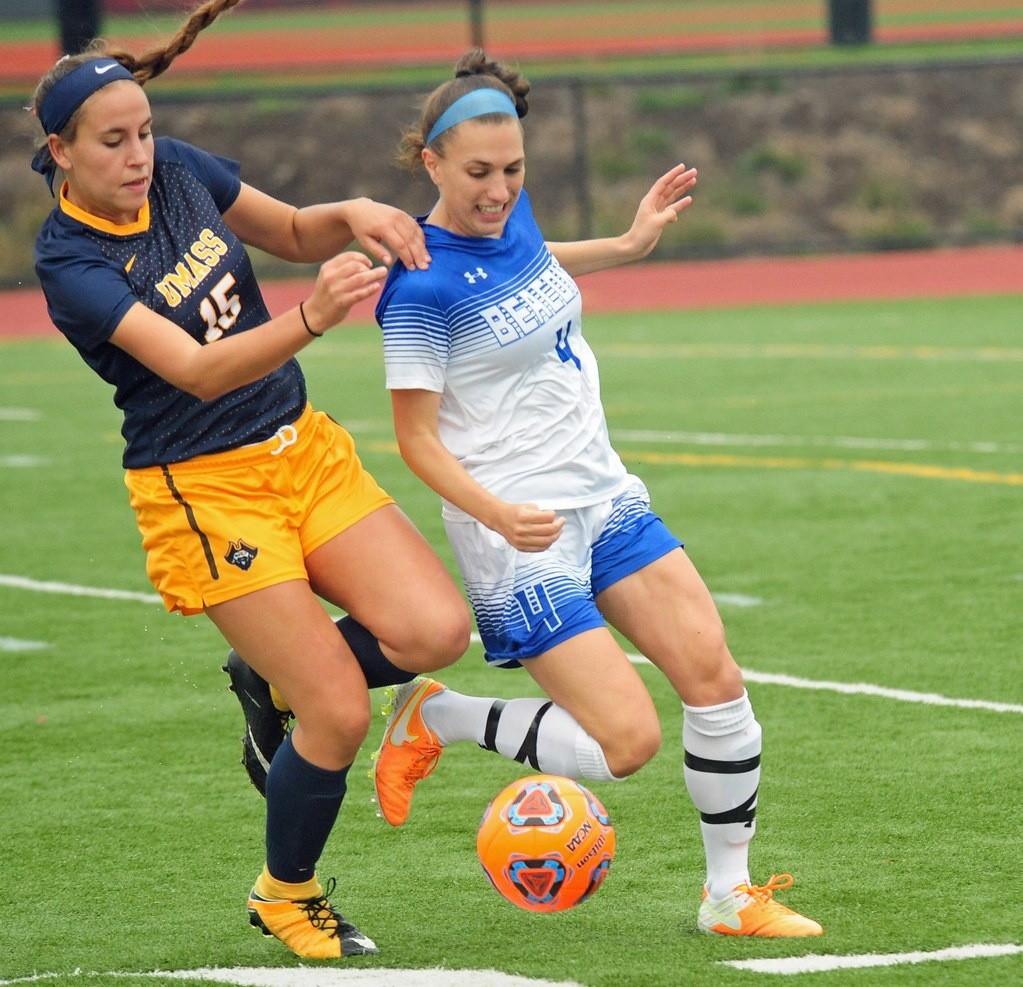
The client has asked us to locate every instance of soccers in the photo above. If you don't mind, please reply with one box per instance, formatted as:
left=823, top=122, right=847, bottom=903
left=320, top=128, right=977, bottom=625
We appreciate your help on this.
left=477, top=774, right=616, bottom=912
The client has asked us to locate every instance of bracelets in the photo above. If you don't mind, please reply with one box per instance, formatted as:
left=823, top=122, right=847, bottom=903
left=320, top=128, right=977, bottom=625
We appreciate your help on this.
left=300, top=302, right=323, bottom=337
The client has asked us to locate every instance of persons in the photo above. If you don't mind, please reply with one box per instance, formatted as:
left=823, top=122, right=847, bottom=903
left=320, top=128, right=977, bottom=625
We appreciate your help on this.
left=25, top=0, right=473, bottom=960
left=367, top=48, right=822, bottom=938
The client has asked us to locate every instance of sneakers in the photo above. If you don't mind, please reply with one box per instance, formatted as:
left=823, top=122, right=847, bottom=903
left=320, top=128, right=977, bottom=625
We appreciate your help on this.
left=696, top=874, right=823, bottom=938
left=373, top=675, right=446, bottom=826
left=246, top=884, right=380, bottom=961
left=222, top=647, right=296, bottom=799
